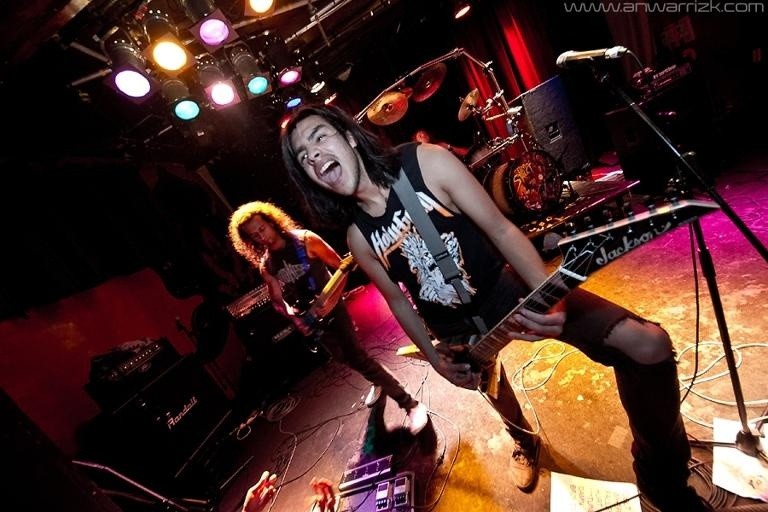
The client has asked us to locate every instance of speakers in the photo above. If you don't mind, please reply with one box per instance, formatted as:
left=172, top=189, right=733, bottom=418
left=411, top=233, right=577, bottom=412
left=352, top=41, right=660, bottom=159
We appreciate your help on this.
left=508, top=73, right=586, bottom=176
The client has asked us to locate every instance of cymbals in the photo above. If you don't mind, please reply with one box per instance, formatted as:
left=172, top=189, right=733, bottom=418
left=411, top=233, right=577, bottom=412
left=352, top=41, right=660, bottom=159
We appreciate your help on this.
left=458, top=88, right=479, bottom=121
left=412, top=63, right=447, bottom=102
left=368, top=92, right=408, bottom=125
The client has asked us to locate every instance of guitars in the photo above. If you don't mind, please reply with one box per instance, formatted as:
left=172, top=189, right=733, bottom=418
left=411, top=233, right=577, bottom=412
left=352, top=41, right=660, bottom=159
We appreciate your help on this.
left=291, top=253, right=359, bottom=355
left=396, top=188, right=720, bottom=399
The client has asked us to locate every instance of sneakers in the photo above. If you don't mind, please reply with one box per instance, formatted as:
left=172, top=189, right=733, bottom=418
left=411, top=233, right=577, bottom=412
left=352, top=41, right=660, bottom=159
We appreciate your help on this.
left=509, top=434, right=540, bottom=489
left=638, top=476, right=714, bottom=512
left=365, top=381, right=383, bottom=407
left=408, top=401, right=428, bottom=436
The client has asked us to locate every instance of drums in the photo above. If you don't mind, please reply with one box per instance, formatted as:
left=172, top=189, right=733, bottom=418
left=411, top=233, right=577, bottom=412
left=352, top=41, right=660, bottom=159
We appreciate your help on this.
left=483, top=149, right=564, bottom=223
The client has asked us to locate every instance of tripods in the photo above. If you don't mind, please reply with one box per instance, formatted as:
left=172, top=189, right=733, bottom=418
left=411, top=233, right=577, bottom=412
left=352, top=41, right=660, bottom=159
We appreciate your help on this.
left=601, top=82, right=768, bottom=463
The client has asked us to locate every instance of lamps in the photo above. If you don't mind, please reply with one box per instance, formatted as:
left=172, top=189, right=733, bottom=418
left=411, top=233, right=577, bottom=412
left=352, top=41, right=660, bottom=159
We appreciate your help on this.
left=103, top=0, right=355, bottom=121
left=453, top=0, right=471, bottom=19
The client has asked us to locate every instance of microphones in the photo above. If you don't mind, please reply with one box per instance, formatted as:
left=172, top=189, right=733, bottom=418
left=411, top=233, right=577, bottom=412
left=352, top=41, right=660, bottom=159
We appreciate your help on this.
left=554, top=44, right=626, bottom=66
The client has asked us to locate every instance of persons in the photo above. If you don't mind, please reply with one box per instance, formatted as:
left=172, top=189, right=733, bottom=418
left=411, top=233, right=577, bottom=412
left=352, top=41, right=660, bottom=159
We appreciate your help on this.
left=281, top=101, right=718, bottom=512
left=241, top=471, right=341, bottom=512
left=223, top=201, right=430, bottom=437
left=411, top=129, right=471, bottom=159
